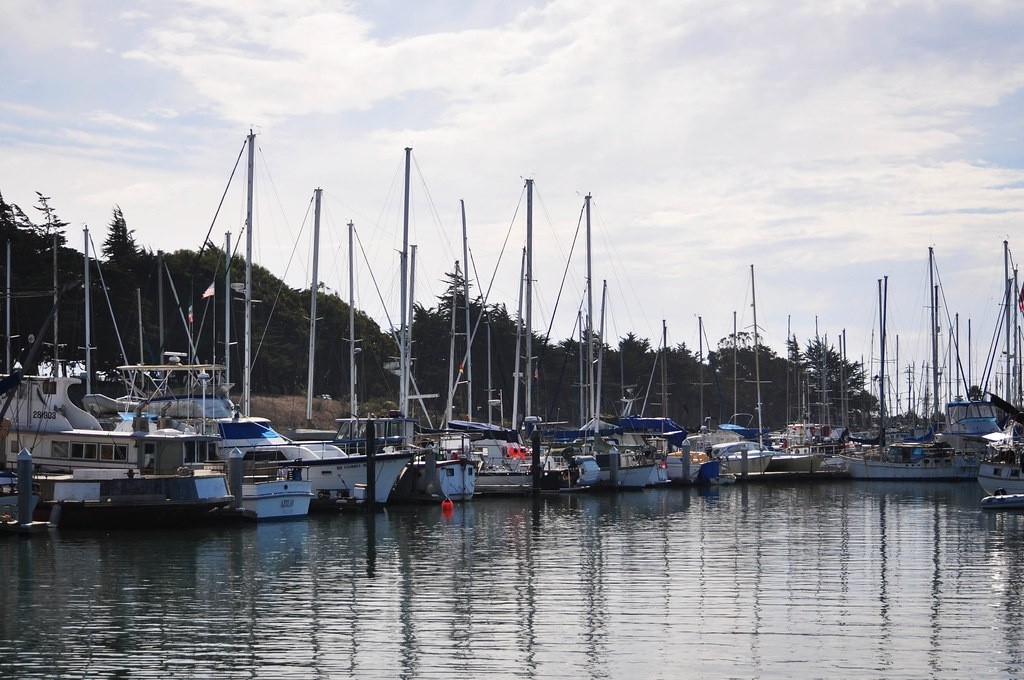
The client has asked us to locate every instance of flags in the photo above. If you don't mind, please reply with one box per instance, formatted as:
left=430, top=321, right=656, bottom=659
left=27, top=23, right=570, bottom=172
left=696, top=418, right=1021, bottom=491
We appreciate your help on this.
left=203, top=282, right=214, bottom=298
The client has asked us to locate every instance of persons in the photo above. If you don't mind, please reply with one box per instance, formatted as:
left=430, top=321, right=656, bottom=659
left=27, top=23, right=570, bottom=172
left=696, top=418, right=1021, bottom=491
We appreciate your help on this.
left=428, top=442, right=440, bottom=459
left=705, top=443, right=713, bottom=458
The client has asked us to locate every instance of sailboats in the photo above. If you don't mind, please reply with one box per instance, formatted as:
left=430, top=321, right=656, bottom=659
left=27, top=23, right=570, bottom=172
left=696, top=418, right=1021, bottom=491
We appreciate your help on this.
left=0, top=128, right=1024, bottom=523
left=974, top=237, right=1024, bottom=523
